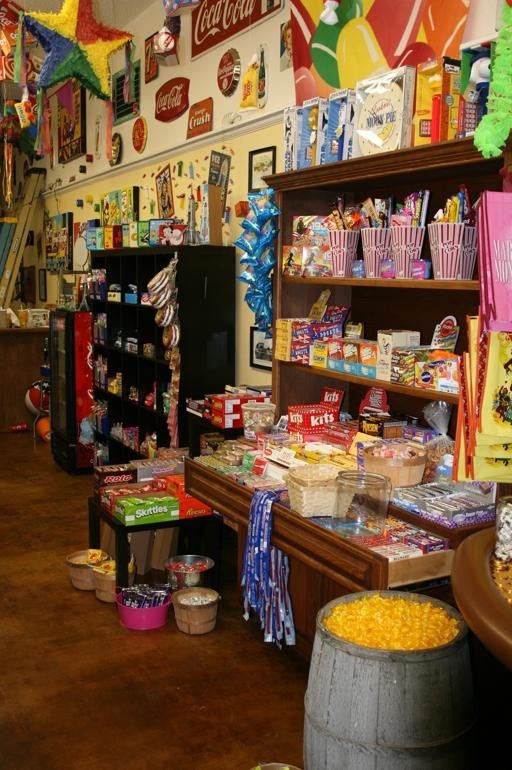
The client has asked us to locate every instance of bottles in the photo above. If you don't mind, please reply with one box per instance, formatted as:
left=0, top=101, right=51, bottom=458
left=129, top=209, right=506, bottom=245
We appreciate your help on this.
left=0, top=306, right=7, bottom=328
left=332, top=470, right=392, bottom=541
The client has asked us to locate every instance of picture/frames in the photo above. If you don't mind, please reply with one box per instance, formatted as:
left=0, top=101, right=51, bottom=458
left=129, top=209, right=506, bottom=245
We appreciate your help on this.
left=248, top=145, right=276, bottom=192
left=38, top=268, right=48, bottom=302
left=247, top=325, right=272, bottom=373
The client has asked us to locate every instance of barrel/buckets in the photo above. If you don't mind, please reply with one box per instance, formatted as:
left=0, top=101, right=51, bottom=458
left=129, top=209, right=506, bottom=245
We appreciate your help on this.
left=302, top=588, right=477, bottom=770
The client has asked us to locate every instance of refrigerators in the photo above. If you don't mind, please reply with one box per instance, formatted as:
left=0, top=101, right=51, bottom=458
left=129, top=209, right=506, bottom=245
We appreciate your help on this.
left=46, top=310, right=93, bottom=475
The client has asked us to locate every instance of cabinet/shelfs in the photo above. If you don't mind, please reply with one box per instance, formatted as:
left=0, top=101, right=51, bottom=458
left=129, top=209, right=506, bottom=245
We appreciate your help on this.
left=184, top=128, right=512, bottom=668
left=91, top=244, right=237, bottom=473
left=186, top=412, right=245, bottom=460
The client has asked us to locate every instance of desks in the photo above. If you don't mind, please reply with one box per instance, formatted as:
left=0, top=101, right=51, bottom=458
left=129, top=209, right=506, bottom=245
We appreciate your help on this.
left=0, top=328, right=57, bottom=434
left=449, top=525, right=512, bottom=672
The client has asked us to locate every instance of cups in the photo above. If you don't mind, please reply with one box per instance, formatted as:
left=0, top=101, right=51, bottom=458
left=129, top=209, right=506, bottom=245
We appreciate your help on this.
left=239, top=402, right=279, bottom=442
left=15, top=310, right=28, bottom=326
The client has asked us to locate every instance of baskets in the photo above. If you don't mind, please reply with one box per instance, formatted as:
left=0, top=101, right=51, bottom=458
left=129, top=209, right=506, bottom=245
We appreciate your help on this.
left=285, top=463, right=353, bottom=518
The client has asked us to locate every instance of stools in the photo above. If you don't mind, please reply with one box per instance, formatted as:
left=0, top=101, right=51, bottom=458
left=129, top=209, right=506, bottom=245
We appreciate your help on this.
left=32, top=364, right=52, bottom=440
left=87, top=498, right=222, bottom=608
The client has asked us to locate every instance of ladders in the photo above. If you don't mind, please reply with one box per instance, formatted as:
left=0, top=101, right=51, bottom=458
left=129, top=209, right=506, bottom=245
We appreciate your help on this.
left=0, top=217, right=17, bottom=280
left=0, top=166, right=46, bottom=309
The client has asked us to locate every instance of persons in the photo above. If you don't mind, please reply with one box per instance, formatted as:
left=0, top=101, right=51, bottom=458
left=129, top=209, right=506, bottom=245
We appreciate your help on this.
left=158, top=176, right=172, bottom=214
left=280, top=20, right=293, bottom=70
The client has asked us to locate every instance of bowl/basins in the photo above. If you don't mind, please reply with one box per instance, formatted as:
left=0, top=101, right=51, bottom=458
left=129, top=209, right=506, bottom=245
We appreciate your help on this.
left=114, top=593, right=173, bottom=629
